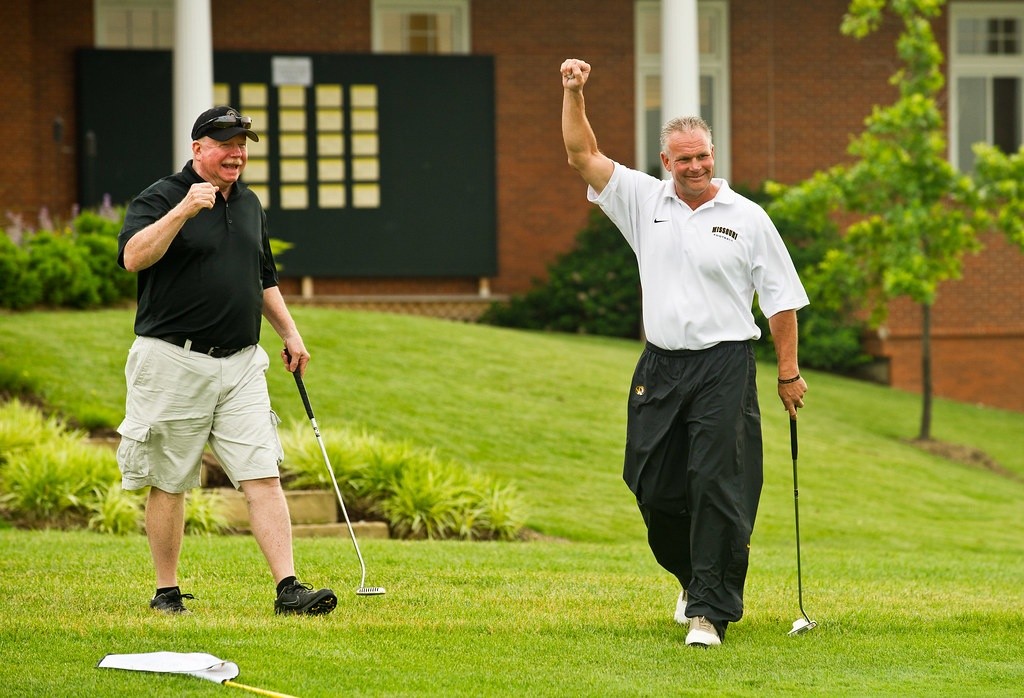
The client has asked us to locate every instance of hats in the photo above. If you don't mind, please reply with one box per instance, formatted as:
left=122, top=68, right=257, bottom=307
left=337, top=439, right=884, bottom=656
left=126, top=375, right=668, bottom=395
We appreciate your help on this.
left=191, top=106, right=259, bottom=142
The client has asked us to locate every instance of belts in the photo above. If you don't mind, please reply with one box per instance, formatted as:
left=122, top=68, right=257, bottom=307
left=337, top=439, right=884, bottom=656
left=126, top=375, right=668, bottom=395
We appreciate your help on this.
left=157, top=335, right=241, bottom=358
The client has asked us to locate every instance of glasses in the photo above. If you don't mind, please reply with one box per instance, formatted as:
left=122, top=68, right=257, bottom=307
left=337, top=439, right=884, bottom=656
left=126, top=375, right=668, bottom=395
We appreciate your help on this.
left=194, top=116, right=252, bottom=134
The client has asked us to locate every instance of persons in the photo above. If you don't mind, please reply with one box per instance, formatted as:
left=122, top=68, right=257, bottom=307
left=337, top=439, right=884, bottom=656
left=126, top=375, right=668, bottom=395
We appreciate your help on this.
left=560, top=58, right=810, bottom=646
left=115, top=106, right=338, bottom=615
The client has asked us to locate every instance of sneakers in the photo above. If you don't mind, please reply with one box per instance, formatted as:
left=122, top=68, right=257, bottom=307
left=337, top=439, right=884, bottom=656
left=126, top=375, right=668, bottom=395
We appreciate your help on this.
left=274, top=580, right=337, bottom=616
left=675, top=590, right=692, bottom=625
left=151, top=589, right=194, bottom=615
left=684, top=616, right=721, bottom=646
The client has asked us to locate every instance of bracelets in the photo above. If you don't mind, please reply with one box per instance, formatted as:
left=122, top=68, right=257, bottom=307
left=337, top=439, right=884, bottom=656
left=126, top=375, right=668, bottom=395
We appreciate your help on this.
left=778, top=374, right=800, bottom=384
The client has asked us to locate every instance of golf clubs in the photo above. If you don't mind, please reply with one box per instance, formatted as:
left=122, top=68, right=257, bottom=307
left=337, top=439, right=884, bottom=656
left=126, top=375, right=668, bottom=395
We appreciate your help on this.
left=785, top=405, right=821, bottom=638
left=283, top=347, right=386, bottom=596
